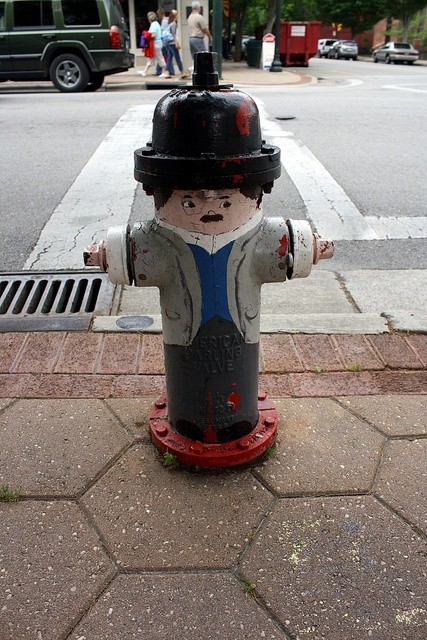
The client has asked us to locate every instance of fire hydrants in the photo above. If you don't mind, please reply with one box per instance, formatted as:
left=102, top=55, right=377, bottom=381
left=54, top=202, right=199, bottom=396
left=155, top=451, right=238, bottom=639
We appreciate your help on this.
left=83, top=49, right=335, bottom=474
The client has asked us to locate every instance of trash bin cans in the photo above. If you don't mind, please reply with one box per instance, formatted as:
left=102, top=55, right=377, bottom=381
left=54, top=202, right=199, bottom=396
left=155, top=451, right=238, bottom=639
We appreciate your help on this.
left=245, top=38, right=261, bottom=65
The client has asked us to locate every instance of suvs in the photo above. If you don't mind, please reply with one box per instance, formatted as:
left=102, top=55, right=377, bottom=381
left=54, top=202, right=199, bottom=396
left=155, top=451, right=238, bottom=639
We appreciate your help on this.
left=328, top=40, right=358, bottom=61
left=0, top=0, right=136, bottom=93
left=315, top=41, right=336, bottom=58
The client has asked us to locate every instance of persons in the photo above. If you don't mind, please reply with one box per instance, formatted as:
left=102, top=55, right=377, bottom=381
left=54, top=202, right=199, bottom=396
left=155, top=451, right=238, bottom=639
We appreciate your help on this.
left=137, top=8, right=190, bottom=80
left=187, top=1, right=213, bottom=75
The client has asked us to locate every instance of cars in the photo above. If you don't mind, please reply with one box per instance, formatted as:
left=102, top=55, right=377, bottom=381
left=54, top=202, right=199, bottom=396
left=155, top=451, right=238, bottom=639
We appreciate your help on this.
left=372, top=42, right=420, bottom=65
